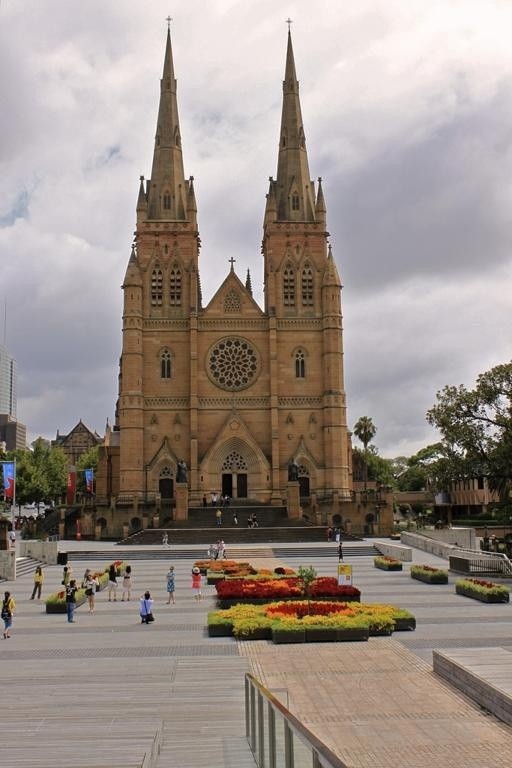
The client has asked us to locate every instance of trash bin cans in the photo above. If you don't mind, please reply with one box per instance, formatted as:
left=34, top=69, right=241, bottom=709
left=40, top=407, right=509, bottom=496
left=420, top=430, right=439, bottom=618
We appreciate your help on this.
left=58, top=551, right=68, bottom=565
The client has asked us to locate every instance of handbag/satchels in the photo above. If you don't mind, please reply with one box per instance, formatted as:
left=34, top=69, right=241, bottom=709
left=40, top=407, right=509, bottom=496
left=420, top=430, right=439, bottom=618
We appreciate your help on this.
left=85, top=588, right=92, bottom=595
left=145, top=614, right=154, bottom=623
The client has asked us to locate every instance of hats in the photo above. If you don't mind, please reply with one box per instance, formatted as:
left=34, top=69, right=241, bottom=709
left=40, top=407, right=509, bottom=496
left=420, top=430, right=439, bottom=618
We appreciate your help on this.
left=193, top=567, right=201, bottom=574
left=70, top=580, right=76, bottom=585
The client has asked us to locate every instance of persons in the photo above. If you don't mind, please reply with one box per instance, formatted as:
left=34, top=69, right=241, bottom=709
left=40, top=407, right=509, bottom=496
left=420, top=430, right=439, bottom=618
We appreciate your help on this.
left=338, top=543, right=344, bottom=563
left=191, top=567, right=202, bottom=604
left=65, top=580, right=77, bottom=622
left=203, top=492, right=259, bottom=529
left=327, top=527, right=341, bottom=542
left=82, top=564, right=132, bottom=614
left=162, top=531, right=170, bottom=548
left=63, top=563, right=73, bottom=590
left=138, top=591, right=154, bottom=624
left=166, top=566, right=176, bottom=605
left=208, top=537, right=227, bottom=560
left=0, top=591, right=15, bottom=639
left=29, top=566, right=43, bottom=599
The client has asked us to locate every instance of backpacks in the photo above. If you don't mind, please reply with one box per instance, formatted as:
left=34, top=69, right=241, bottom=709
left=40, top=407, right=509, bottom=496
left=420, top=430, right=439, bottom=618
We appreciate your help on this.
left=2, top=599, right=13, bottom=622
